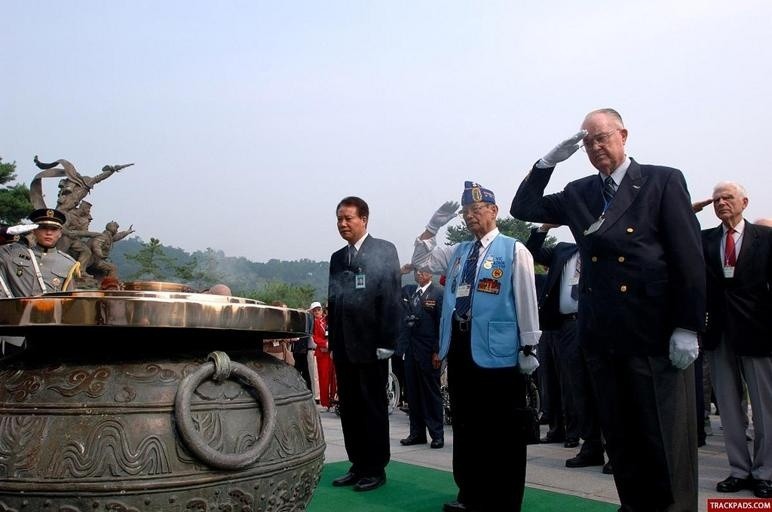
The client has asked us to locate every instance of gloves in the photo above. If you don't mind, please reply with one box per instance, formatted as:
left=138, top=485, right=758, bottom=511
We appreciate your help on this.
left=377, top=348, right=394, bottom=360
left=668, top=327, right=699, bottom=370
left=540, top=129, right=589, bottom=167
left=6, top=224, right=37, bottom=236
left=518, top=349, right=538, bottom=369
left=425, top=200, right=460, bottom=235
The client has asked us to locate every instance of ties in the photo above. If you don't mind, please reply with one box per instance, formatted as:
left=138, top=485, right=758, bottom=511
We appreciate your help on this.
left=725, top=229, right=737, bottom=268
left=604, top=176, right=616, bottom=204
left=349, top=244, right=357, bottom=265
left=571, top=256, right=581, bottom=301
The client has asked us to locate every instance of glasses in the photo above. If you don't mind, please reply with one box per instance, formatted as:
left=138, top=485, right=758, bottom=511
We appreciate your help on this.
left=580, top=133, right=614, bottom=152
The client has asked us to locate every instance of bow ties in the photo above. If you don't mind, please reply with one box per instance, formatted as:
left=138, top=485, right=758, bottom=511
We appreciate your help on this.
left=456, top=240, right=482, bottom=317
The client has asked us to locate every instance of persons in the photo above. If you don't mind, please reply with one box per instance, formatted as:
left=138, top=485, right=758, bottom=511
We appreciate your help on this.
left=0, top=208, right=82, bottom=299
left=510, top=109, right=708, bottom=512
left=55, top=163, right=136, bottom=279
left=207, top=181, right=771, bottom=512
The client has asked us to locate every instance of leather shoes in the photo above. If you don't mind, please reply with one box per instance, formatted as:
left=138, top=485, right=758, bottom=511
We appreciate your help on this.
left=541, top=437, right=579, bottom=447
left=400, top=435, right=444, bottom=448
left=443, top=501, right=467, bottom=511
left=333, top=473, right=386, bottom=492
left=717, top=477, right=772, bottom=499
left=566, top=455, right=614, bottom=474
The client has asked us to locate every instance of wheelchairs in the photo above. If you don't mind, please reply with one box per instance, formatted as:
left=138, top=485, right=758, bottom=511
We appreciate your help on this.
left=397, top=367, right=452, bottom=427
left=524, top=377, right=543, bottom=423
left=329, top=356, right=401, bottom=418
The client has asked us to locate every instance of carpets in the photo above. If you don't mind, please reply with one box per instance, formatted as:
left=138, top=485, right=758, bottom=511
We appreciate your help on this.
left=299, top=454, right=621, bottom=511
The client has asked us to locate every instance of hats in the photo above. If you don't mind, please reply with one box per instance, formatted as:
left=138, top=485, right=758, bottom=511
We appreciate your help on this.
left=309, top=302, right=321, bottom=311
left=462, top=181, right=495, bottom=205
left=29, top=207, right=66, bottom=232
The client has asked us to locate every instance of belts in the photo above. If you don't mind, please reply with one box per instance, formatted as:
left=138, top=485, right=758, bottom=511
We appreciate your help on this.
left=308, top=348, right=314, bottom=350
